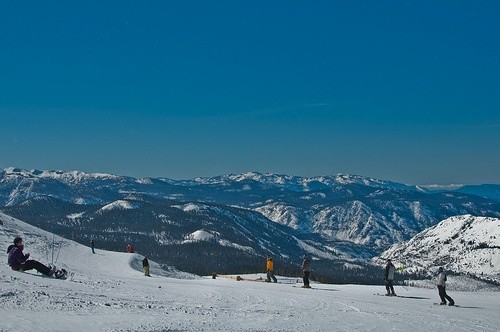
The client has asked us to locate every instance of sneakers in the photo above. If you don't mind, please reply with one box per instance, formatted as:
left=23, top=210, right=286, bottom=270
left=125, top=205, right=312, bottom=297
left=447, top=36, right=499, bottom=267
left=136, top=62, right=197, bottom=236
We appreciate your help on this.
left=448, top=299, right=454, bottom=306
left=391, top=290, right=396, bottom=296
left=440, top=299, right=446, bottom=305
left=48, top=265, right=56, bottom=276
left=385, top=290, right=391, bottom=295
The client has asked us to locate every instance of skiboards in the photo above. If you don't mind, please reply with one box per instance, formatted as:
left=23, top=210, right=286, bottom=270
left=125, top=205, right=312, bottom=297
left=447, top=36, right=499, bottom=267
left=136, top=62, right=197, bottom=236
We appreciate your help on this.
left=373, top=293, right=406, bottom=298
left=433, top=303, right=460, bottom=307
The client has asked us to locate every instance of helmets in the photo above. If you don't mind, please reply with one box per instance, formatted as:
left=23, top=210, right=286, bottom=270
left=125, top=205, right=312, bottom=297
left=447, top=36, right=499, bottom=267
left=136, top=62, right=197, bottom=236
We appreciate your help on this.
left=55, top=269, right=67, bottom=280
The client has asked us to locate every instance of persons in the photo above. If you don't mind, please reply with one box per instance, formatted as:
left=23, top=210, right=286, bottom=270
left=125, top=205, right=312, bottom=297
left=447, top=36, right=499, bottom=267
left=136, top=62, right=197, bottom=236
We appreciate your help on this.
left=265, top=254, right=277, bottom=283
left=143, top=255, right=151, bottom=276
left=436, top=267, right=454, bottom=306
left=301, top=254, right=311, bottom=288
left=385, top=259, right=396, bottom=296
left=89, top=239, right=97, bottom=254
left=7, top=236, right=56, bottom=276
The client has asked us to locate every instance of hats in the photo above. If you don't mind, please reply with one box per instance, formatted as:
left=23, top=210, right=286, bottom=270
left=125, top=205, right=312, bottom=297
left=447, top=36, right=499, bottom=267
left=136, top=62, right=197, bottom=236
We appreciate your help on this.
left=303, top=255, right=307, bottom=258
